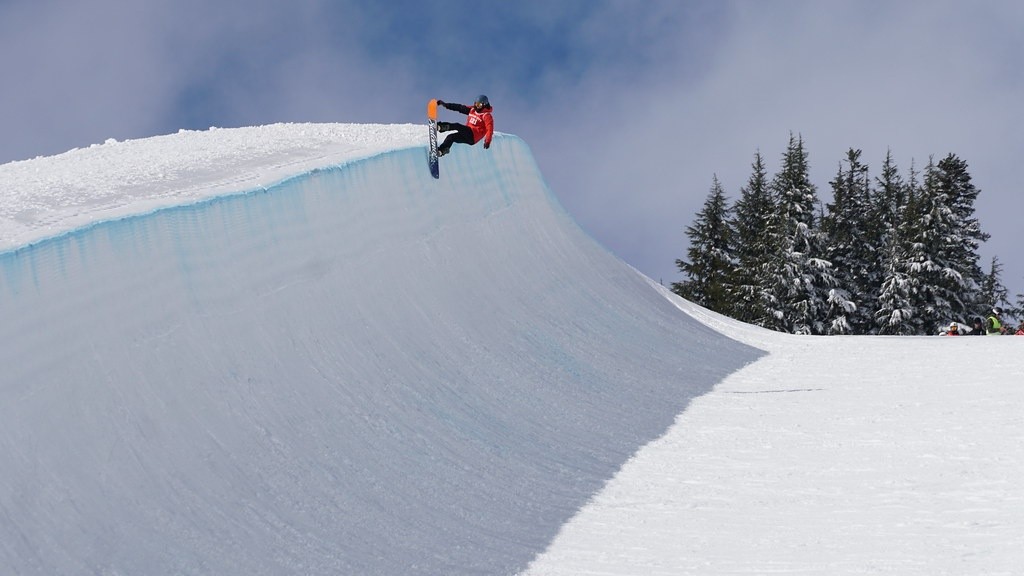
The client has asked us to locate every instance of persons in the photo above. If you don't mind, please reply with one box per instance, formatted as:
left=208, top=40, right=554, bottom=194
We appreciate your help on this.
left=437, top=94, right=494, bottom=157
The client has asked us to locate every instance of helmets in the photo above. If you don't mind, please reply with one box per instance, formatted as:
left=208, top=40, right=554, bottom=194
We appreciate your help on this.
left=474, top=95, right=488, bottom=111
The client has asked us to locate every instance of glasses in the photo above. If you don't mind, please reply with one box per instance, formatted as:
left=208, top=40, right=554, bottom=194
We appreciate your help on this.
left=475, top=102, right=488, bottom=107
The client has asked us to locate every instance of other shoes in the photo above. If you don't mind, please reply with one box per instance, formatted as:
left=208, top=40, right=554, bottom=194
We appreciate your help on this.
left=437, top=121, right=445, bottom=133
left=437, top=147, right=443, bottom=157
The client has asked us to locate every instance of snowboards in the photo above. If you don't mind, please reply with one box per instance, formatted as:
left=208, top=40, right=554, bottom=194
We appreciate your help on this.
left=424, top=100, right=444, bottom=183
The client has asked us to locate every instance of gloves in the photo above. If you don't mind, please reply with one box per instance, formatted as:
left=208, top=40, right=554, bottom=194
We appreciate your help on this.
left=484, top=142, right=489, bottom=149
left=436, top=100, right=445, bottom=106
